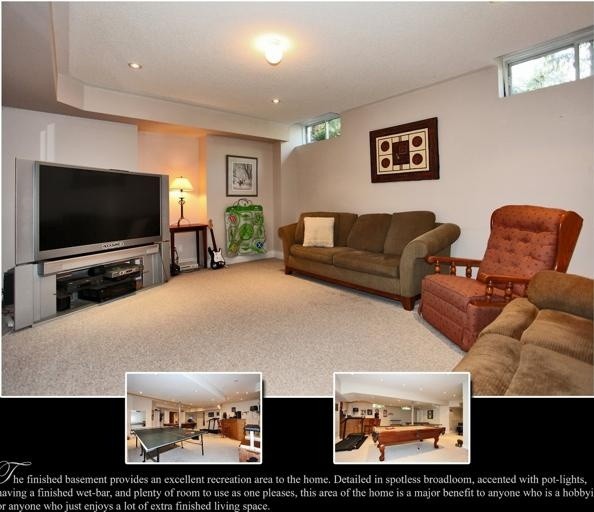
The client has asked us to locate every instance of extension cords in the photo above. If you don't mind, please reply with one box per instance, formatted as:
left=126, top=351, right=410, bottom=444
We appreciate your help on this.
left=7, top=313, right=15, bottom=328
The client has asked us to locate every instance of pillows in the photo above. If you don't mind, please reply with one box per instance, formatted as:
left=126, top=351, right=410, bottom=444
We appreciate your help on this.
left=383, top=211, right=436, bottom=256
left=346, top=214, right=391, bottom=253
left=295, top=213, right=339, bottom=245
left=335, top=214, right=358, bottom=248
left=302, top=216, right=336, bottom=248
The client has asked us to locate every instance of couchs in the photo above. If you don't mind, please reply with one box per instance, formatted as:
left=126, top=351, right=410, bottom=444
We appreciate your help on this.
left=278, top=211, right=461, bottom=312
left=418, top=204, right=584, bottom=353
left=450, top=270, right=593, bottom=397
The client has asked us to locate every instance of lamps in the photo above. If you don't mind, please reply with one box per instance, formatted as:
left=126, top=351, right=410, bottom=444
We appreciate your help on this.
left=170, top=176, right=195, bottom=226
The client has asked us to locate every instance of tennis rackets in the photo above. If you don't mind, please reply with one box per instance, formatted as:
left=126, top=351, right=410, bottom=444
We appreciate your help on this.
left=183, top=430, right=195, bottom=435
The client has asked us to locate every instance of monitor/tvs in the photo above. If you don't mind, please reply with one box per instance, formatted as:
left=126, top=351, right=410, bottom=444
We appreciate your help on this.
left=15, top=159, right=170, bottom=265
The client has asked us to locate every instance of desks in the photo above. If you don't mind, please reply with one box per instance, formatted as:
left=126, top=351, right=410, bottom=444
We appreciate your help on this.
left=133, top=427, right=208, bottom=462
left=169, top=223, right=209, bottom=274
left=372, top=425, right=446, bottom=461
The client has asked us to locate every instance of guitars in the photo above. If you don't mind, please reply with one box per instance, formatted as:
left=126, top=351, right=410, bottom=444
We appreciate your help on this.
left=207, top=220, right=226, bottom=269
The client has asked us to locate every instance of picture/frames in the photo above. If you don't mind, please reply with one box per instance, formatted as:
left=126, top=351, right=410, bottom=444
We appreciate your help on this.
left=368, top=116, right=440, bottom=183
left=225, top=154, right=259, bottom=198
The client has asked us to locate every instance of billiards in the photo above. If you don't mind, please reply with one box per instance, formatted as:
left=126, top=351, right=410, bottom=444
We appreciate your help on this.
left=385, top=426, right=396, bottom=430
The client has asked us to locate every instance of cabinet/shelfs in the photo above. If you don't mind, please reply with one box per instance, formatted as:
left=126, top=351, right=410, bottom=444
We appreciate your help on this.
left=220, top=419, right=246, bottom=442
left=238, top=424, right=261, bottom=462
left=51, top=254, right=147, bottom=312
